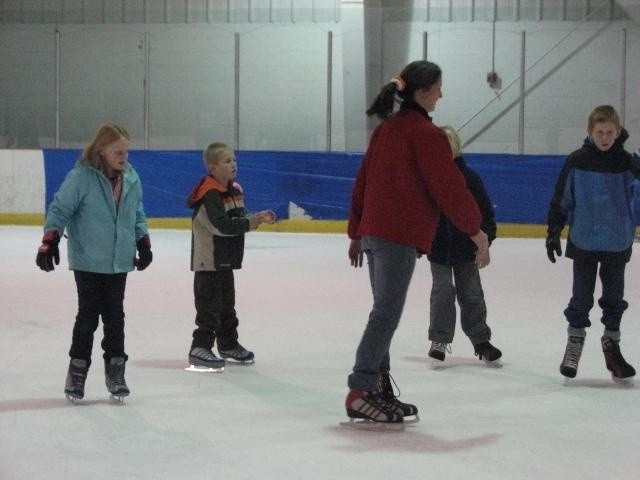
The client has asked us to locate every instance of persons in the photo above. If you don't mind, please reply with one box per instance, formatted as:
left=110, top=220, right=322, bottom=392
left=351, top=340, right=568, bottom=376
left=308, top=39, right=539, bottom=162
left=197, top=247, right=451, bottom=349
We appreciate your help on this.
left=416, top=125, right=501, bottom=361
left=36, top=122, right=153, bottom=399
left=345, top=60, right=489, bottom=423
left=546, top=105, right=640, bottom=379
left=184, top=143, right=276, bottom=369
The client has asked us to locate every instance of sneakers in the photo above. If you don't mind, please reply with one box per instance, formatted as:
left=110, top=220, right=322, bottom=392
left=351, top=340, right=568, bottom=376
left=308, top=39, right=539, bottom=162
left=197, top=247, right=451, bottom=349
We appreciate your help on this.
left=189, top=347, right=224, bottom=367
left=218, top=345, right=254, bottom=361
left=427, top=341, right=452, bottom=361
left=473, top=342, right=502, bottom=361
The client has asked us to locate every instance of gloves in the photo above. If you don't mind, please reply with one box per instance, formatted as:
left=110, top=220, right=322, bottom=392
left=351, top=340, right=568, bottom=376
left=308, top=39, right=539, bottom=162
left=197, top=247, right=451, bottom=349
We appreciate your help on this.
left=545, top=233, right=562, bottom=263
left=36, top=230, right=60, bottom=271
left=134, top=235, right=152, bottom=270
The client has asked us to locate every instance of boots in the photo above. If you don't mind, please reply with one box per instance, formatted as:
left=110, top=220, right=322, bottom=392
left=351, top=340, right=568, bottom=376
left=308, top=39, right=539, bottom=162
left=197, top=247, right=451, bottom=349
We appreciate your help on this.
left=559, top=336, right=584, bottom=378
left=104, top=356, right=130, bottom=396
left=64, top=358, right=91, bottom=398
left=345, top=371, right=418, bottom=423
left=600, top=336, right=635, bottom=379
